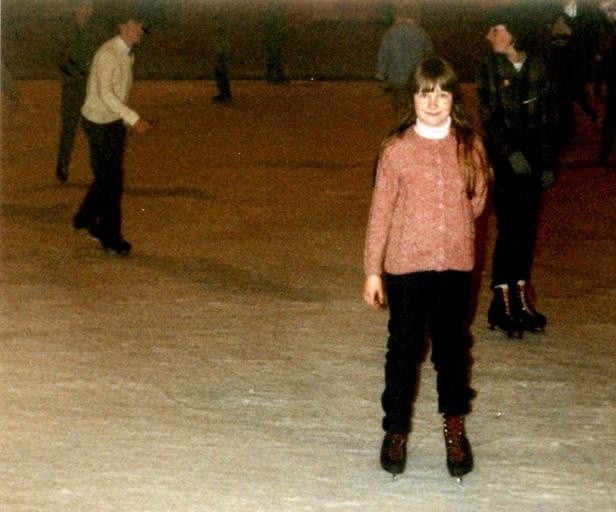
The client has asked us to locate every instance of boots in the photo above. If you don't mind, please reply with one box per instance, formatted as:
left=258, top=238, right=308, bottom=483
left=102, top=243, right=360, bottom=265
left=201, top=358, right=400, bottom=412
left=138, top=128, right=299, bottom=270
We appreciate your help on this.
left=443, top=416, right=472, bottom=476
left=381, top=432, right=406, bottom=473
left=487, top=280, right=546, bottom=332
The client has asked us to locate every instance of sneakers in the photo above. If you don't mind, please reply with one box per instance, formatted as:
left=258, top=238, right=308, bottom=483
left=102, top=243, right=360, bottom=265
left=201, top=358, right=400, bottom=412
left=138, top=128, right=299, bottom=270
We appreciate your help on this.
left=72, top=213, right=131, bottom=253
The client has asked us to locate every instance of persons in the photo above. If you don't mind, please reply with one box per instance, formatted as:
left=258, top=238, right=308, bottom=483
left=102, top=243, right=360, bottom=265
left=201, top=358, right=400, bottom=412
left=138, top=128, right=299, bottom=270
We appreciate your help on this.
left=55, top=2, right=113, bottom=184
left=530, top=0, right=614, bottom=168
left=257, top=2, right=289, bottom=82
left=481, top=14, right=561, bottom=340
left=371, top=1, right=434, bottom=118
left=362, top=55, right=492, bottom=478
left=209, top=2, right=234, bottom=104
left=71, top=12, right=150, bottom=258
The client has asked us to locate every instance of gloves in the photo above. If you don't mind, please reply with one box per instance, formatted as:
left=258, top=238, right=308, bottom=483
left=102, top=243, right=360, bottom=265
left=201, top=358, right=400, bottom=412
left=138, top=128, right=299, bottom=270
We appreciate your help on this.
left=538, top=169, right=555, bottom=186
left=508, top=151, right=530, bottom=173
